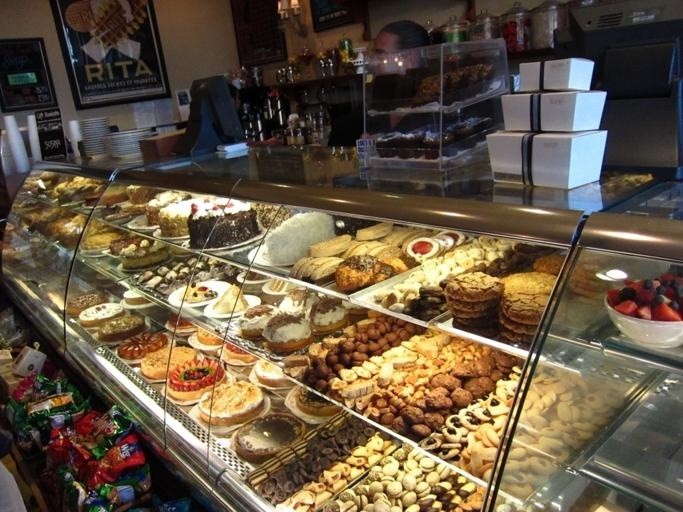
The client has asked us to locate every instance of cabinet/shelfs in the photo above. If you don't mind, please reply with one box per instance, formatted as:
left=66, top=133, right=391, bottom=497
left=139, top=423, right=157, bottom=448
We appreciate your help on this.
left=0, top=149, right=683, bottom=511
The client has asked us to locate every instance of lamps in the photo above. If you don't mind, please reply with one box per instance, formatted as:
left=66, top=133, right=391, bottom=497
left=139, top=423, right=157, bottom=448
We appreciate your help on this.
left=275, top=0, right=307, bottom=39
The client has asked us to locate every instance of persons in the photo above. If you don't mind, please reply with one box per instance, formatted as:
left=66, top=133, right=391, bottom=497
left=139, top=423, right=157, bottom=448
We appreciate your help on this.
left=325, top=19, right=448, bottom=149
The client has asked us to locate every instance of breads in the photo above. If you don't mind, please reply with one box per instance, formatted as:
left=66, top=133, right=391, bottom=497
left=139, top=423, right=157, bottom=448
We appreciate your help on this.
left=290, top=221, right=473, bottom=393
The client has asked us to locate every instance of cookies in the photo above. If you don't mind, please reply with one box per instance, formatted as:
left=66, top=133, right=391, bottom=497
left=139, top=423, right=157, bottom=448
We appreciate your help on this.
left=257, top=253, right=625, bottom=512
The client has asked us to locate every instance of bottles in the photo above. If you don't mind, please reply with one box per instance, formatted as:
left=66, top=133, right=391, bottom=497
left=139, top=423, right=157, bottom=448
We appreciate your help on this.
left=228, top=34, right=367, bottom=148
left=418, top=1, right=566, bottom=55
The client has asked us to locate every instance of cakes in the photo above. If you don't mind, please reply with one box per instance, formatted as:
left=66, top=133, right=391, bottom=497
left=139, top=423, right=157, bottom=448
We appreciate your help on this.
left=78, top=189, right=368, bottom=464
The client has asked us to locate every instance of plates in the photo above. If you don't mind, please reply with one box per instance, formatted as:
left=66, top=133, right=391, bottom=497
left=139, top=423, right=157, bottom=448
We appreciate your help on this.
left=81, top=114, right=146, bottom=163
left=62, top=195, right=340, bottom=468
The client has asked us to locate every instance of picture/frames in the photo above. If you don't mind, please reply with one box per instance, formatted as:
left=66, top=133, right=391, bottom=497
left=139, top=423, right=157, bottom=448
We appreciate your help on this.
left=50, top=1, right=172, bottom=112
left=229, top=1, right=288, bottom=68
left=0, top=37, right=59, bottom=115
left=308, top=0, right=365, bottom=34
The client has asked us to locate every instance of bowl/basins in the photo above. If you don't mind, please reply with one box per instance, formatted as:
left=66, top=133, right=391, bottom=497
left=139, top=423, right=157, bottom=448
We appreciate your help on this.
left=604, top=286, right=683, bottom=349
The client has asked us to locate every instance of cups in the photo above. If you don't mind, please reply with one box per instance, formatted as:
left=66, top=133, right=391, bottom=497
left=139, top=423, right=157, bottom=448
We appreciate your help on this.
left=0, top=114, right=81, bottom=176
